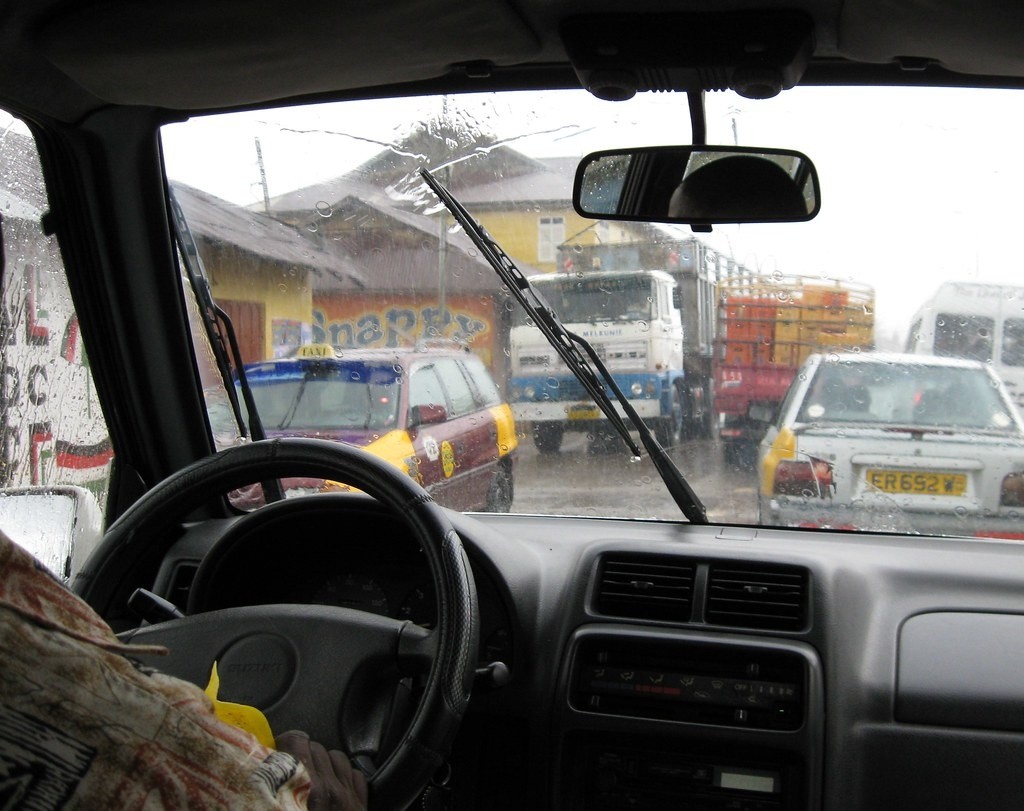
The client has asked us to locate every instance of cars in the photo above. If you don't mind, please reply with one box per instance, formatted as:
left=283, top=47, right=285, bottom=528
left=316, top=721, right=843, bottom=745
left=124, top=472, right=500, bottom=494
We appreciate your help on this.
left=746, top=351, right=1024, bottom=539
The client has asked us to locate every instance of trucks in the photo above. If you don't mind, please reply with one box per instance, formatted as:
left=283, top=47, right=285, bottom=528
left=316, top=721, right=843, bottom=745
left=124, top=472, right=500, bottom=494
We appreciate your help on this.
left=505, top=269, right=717, bottom=463
left=709, top=275, right=878, bottom=454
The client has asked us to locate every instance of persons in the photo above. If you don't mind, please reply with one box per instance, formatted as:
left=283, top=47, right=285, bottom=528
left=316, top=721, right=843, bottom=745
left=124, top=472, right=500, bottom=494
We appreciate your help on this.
left=669, top=156, right=808, bottom=219
left=1, top=530, right=368, bottom=811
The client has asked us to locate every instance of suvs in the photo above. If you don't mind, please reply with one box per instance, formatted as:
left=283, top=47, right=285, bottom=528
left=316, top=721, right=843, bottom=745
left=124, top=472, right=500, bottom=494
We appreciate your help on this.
left=213, top=337, right=519, bottom=513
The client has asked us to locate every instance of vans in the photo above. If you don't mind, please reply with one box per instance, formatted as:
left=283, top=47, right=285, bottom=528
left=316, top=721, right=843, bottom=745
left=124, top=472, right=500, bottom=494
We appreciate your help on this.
left=903, top=280, right=1024, bottom=412
